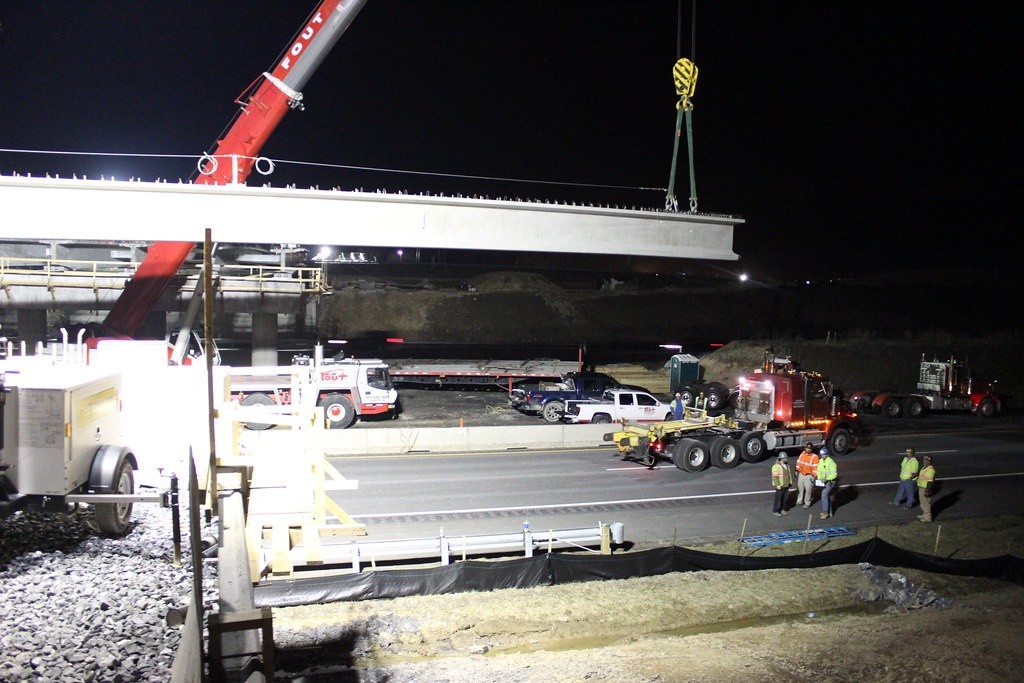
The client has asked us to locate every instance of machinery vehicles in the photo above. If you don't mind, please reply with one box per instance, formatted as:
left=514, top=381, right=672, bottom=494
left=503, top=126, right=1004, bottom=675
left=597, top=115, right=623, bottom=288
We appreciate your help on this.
left=227, top=352, right=399, bottom=432
left=1, top=0, right=697, bottom=538
left=599, top=352, right=860, bottom=476
left=849, top=354, right=1002, bottom=431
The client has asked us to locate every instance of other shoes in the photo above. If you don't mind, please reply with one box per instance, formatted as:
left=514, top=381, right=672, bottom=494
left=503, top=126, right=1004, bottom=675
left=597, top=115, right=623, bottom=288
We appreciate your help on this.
left=772, top=512, right=782, bottom=517
left=901, top=505, right=911, bottom=510
left=917, top=514, right=932, bottom=518
left=803, top=504, right=810, bottom=508
left=781, top=510, right=788, bottom=515
left=821, top=513, right=830, bottom=519
left=820, top=512, right=832, bottom=517
left=794, top=502, right=803, bottom=505
left=889, top=501, right=900, bottom=506
left=920, top=518, right=932, bottom=522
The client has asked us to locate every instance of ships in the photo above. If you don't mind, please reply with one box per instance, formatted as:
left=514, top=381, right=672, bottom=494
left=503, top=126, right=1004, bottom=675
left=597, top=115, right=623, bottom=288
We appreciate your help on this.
left=270, top=243, right=380, bottom=273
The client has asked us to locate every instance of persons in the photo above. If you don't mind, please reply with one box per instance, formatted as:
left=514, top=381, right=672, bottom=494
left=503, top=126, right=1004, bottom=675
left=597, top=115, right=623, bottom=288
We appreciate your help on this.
left=771, top=452, right=793, bottom=517
left=816, top=447, right=838, bottom=520
left=670, top=392, right=686, bottom=421
left=692, top=390, right=710, bottom=416
left=916, top=455, right=936, bottom=523
left=889, top=447, right=919, bottom=510
left=794, top=442, right=820, bottom=509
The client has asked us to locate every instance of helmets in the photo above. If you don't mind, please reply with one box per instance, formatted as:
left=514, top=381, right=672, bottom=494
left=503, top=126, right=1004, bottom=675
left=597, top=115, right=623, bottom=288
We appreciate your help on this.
left=778, top=451, right=788, bottom=460
left=905, top=447, right=915, bottom=453
left=675, top=392, right=681, bottom=397
left=806, top=442, right=812, bottom=449
left=819, top=447, right=829, bottom=455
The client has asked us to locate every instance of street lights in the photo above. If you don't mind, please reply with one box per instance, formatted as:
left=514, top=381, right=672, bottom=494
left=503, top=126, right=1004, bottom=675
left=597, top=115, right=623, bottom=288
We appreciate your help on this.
left=398, top=250, right=403, bottom=261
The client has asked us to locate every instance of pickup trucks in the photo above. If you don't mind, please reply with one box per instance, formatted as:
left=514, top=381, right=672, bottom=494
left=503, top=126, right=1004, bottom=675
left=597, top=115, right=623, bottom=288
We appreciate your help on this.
left=510, top=371, right=652, bottom=422
left=554, top=388, right=672, bottom=425
